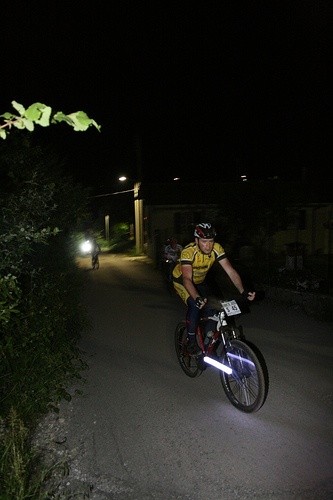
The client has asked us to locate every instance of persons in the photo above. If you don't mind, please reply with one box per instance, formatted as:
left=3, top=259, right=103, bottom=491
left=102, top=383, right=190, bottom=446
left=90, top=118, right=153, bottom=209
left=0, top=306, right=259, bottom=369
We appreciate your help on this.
left=164, top=238, right=183, bottom=264
left=171, top=223, right=256, bottom=356
left=92, top=239, right=100, bottom=269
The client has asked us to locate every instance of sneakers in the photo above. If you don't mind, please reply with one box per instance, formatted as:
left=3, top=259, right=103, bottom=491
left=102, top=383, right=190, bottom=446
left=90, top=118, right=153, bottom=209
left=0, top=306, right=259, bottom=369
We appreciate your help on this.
left=184, top=343, right=203, bottom=357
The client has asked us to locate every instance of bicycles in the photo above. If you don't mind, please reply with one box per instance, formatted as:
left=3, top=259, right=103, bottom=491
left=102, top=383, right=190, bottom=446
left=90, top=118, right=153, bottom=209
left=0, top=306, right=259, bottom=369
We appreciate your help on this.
left=170, top=283, right=271, bottom=414
left=88, top=246, right=101, bottom=270
left=163, top=258, right=180, bottom=282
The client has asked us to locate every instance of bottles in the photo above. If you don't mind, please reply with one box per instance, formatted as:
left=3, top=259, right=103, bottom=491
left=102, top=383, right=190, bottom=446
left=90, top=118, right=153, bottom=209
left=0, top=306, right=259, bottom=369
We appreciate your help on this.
left=202, top=329, right=214, bottom=346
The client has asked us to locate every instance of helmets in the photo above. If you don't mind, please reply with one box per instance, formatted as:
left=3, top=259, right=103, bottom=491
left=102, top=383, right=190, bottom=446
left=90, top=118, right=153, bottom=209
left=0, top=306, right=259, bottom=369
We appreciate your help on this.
left=194, top=222, right=215, bottom=239
